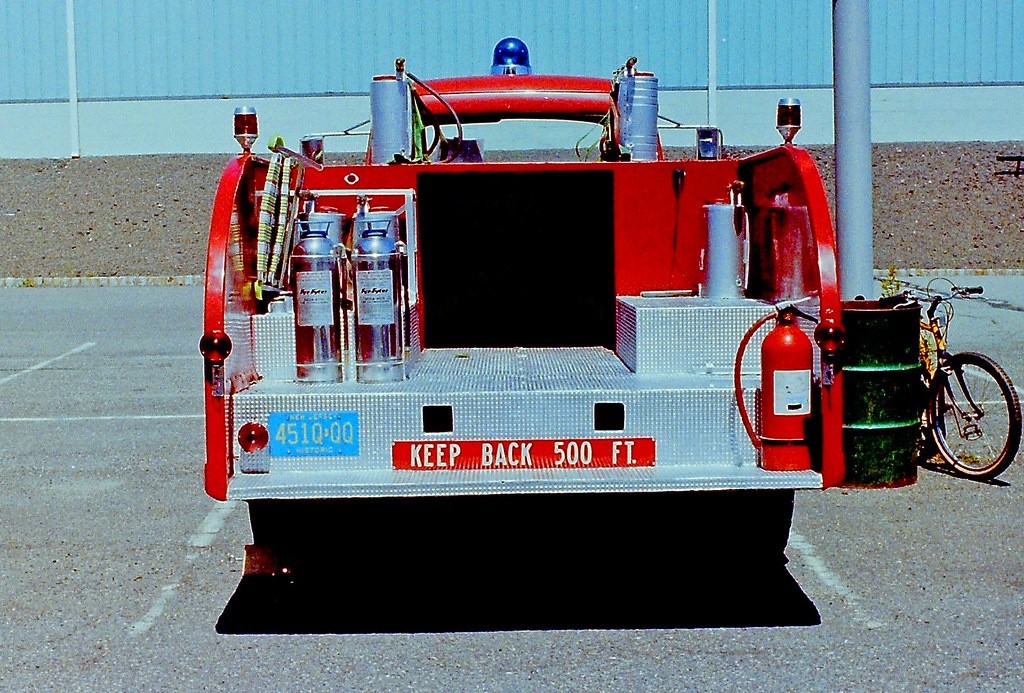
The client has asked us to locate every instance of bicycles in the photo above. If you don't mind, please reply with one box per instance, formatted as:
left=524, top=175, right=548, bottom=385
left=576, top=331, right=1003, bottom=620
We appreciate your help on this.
left=878, top=277, right=1022, bottom=483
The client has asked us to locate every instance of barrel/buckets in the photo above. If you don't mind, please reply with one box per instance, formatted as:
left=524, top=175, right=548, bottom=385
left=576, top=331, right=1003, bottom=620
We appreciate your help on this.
left=840, top=298, right=923, bottom=488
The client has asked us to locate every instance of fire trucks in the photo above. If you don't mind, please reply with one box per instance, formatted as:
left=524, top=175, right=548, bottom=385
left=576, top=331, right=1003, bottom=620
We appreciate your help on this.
left=197, top=32, right=844, bottom=558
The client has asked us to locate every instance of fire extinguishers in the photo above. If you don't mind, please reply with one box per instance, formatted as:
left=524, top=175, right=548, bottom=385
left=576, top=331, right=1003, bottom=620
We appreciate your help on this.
left=731, top=296, right=819, bottom=472
